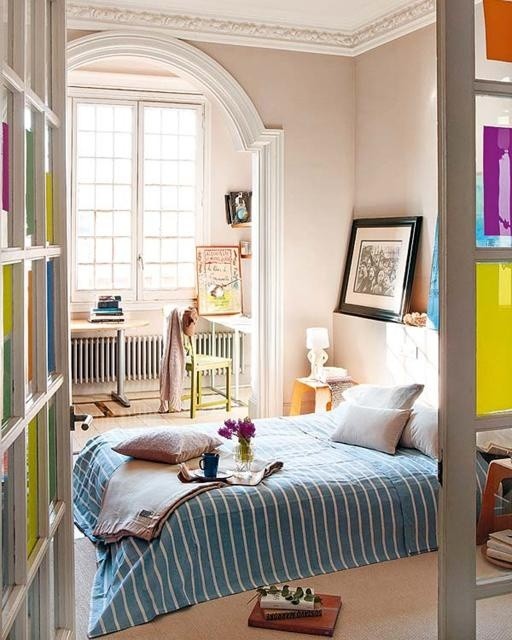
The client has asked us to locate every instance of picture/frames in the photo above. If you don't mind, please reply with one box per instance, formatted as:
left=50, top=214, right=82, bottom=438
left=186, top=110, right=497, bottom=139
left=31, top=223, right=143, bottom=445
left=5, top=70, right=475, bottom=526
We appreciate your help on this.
left=336, top=215, right=423, bottom=323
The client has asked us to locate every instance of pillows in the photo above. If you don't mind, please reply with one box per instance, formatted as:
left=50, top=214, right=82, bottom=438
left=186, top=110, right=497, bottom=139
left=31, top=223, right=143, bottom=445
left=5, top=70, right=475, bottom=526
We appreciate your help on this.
left=111, top=426, right=212, bottom=463
left=328, top=404, right=412, bottom=455
left=402, top=401, right=439, bottom=459
left=341, top=381, right=423, bottom=413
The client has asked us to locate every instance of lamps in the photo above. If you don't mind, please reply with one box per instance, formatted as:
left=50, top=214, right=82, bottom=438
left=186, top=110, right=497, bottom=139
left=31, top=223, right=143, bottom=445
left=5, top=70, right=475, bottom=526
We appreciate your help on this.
left=304, top=327, right=330, bottom=381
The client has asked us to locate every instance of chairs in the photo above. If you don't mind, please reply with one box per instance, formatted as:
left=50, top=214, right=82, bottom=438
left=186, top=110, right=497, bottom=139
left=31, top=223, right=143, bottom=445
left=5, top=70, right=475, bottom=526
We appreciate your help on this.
left=174, top=310, right=232, bottom=419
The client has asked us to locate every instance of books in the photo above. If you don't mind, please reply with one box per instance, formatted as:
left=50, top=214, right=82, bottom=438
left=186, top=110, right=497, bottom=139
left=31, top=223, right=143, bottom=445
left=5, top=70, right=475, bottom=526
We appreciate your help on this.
left=87, top=293, right=127, bottom=324
left=485, top=527, right=511, bottom=563
left=247, top=581, right=344, bottom=636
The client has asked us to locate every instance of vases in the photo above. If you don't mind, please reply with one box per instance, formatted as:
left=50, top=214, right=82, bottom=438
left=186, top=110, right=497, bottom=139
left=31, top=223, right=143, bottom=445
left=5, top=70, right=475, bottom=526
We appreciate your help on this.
left=234, top=440, right=253, bottom=467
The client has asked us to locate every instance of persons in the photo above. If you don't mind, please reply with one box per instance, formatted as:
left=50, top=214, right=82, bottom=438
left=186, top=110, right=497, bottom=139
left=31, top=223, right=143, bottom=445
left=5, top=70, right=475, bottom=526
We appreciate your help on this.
left=234, top=191, right=249, bottom=223
left=351, top=244, right=402, bottom=295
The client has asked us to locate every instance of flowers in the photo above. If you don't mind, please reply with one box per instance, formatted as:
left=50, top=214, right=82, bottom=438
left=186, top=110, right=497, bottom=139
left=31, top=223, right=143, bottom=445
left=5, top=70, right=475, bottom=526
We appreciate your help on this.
left=219, top=415, right=258, bottom=445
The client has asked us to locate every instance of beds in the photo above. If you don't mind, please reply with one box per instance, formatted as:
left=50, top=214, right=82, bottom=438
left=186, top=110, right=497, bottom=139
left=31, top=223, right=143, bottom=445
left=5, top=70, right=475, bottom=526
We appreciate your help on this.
left=71, top=409, right=505, bottom=638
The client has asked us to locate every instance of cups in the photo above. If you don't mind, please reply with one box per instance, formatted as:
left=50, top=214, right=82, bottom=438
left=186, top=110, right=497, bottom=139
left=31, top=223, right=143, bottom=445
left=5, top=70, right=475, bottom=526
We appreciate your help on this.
left=240, top=240, right=249, bottom=258
left=199, top=453, right=221, bottom=477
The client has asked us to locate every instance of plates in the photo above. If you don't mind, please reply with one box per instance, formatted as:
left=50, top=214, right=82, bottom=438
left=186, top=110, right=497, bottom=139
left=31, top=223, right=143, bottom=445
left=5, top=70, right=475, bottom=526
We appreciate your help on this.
left=194, top=469, right=233, bottom=480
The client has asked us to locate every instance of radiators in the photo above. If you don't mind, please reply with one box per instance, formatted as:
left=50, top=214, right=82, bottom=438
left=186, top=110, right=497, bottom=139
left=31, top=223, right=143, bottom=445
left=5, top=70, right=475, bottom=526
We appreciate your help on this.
left=71, top=331, right=246, bottom=383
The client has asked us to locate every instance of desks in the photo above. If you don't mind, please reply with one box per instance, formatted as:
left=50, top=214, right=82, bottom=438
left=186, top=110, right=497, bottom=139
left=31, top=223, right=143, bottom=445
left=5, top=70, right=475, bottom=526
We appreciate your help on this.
left=476, top=459, right=512, bottom=545
left=202, top=313, right=253, bottom=408
left=289, top=379, right=351, bottom=416
left=70, top=317, right=151, bottom=407
